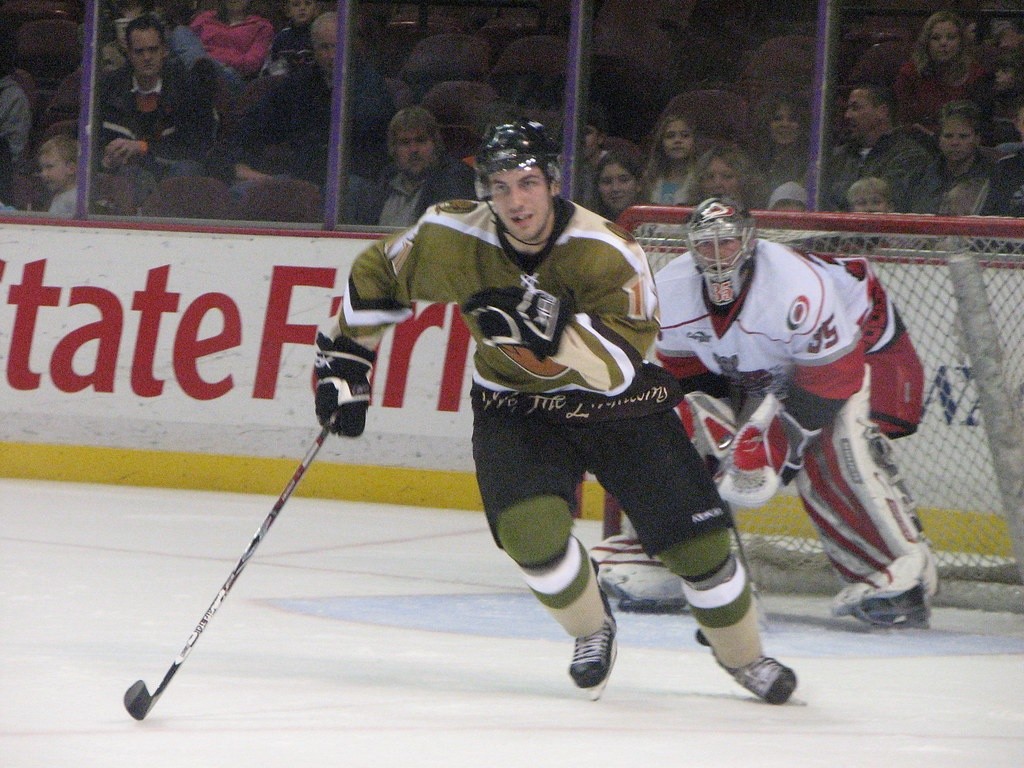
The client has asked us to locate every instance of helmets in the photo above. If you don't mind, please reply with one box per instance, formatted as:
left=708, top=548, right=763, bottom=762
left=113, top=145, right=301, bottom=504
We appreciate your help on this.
left=685, top=198, right=752, bottom=304
left=474, top=120, right=559, bottom=198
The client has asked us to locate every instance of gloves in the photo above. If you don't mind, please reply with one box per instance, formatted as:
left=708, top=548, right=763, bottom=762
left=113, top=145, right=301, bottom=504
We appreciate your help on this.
left=309, top=340, right=375, bottom=437
left=463, top=284, right=566, bottom=362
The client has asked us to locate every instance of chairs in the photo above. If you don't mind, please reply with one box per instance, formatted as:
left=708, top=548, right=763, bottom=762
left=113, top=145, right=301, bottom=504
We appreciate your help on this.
left=0, top=0, right=1024, bottom=225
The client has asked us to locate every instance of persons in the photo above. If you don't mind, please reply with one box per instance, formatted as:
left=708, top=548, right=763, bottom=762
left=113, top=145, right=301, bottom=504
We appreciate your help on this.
left=312, top=118, right=800, bottom=706
left=595, top=196, right=929, bottom=615
left=0, top=0, right=1024, bottom=218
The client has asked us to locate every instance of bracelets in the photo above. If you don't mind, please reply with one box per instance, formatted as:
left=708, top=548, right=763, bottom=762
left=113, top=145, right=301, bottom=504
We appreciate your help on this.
left=139, top=140, right=149, bottom=155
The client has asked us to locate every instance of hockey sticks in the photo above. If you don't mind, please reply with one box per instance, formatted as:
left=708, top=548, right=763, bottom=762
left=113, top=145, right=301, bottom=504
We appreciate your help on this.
left=123, top=397, right=343, bottom=724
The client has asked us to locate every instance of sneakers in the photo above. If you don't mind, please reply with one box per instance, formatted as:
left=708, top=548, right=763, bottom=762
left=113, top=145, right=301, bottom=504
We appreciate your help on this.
left=855, top=589, right=931, bottom=631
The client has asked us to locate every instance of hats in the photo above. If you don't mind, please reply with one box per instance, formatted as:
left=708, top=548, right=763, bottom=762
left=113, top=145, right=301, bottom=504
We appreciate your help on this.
left=770, top=181, right=809, bottom=212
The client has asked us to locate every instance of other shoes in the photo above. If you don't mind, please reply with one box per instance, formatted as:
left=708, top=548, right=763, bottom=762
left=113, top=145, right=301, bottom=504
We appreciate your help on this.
left=728, top=654, right=798, bottom=704
left=568, top=602, right=618, bottom=698
